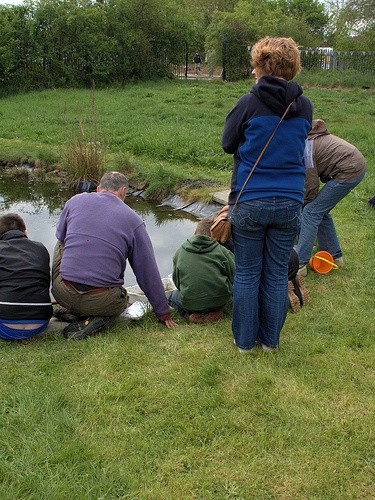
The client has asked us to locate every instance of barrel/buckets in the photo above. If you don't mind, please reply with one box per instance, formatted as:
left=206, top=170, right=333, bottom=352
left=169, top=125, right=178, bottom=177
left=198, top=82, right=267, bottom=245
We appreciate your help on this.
left=308, top=251, right=339, bottom=275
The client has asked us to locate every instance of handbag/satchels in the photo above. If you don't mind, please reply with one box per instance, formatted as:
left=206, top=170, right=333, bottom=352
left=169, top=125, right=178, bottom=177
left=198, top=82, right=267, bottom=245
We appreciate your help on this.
left=210, top=205, right=232, bottom=243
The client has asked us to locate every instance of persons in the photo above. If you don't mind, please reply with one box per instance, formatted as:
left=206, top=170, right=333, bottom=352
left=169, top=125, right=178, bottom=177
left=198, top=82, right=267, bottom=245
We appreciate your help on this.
left=0, top=212, right=54, bottom=341
left=167, top=218, right=309, bottom=323
left=193, top=50, right=202, bottom=75
left=209, top=35, right=312, bottom=355
left=51, top=170, right=180, bottom=341
left=297, top=118, right=366, bottom=277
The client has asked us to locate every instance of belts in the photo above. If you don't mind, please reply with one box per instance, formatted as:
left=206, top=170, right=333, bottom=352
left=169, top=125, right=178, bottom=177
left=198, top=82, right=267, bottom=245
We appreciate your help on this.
left=63, top=280, right=109, bottom=295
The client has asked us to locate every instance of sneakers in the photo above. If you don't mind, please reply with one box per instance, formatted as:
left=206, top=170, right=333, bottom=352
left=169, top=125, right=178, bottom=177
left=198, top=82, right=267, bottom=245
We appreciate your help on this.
left=293, top=276, right=310, bottom=306
left=288, top=281, right=300, bottom=312
left=53, top=307, right=80, bottom=322
left=189, top=309, right=223, bottom=323
left=63, top=316, right=107, bottom=339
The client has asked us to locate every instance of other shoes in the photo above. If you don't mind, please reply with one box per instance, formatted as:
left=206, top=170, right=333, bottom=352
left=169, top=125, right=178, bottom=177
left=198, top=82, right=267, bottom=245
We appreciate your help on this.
left=263, top=343, right=278, bottom=353
left=234, top=339, right=253, bottom=353
left=335, top=257, right=343, bottom=266
left=298, top=265, right=308, bottom=277
